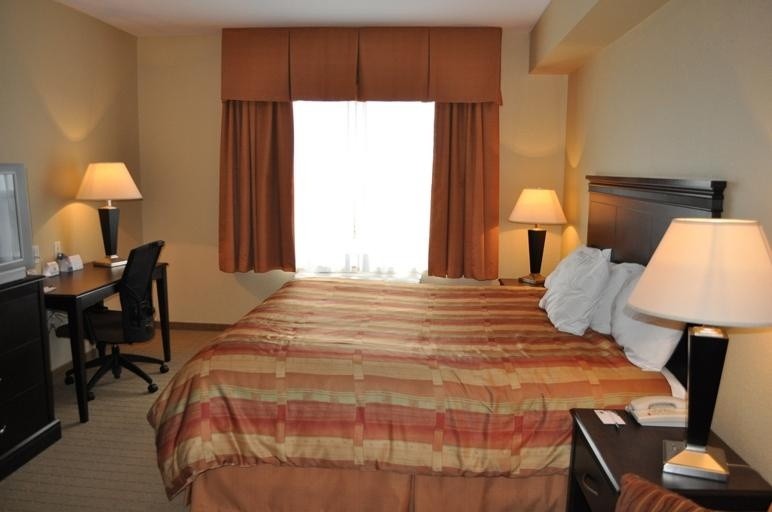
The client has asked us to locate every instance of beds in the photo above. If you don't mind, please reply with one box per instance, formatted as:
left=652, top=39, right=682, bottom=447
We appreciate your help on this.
left=180, top=179, right=723, bottom=512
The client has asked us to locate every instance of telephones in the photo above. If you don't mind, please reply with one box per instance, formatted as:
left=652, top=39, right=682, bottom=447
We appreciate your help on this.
left=628, top=395, right=688, bottom=427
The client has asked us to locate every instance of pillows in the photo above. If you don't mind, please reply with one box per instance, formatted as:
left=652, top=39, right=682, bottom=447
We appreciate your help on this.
left=539, top=246, right=685, bottom=374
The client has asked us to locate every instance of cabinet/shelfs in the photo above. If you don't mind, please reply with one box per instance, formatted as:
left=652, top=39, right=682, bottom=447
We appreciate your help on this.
left=0, top=273, right=64, bottom=481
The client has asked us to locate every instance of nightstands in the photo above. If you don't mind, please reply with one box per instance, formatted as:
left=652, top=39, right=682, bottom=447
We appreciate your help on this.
left=563, top=407, right=770, bottom=512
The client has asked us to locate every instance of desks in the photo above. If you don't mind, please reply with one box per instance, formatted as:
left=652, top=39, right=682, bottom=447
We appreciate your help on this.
left=42, top=264, right=168, bottom=423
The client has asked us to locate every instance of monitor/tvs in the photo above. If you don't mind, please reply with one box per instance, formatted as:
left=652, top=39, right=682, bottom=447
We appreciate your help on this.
left=0, top=163, right=35, bottom=284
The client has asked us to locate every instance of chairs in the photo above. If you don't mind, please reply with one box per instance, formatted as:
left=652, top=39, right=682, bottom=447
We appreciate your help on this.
left=54, top=238, right=171, bottom=400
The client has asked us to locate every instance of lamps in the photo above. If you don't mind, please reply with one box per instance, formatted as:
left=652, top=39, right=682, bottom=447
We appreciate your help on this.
left=626, top=213, right=772, bottom=485
left=508, top=189, right=570, bottom=286
left=75, top=162, right=146, bottom=268
